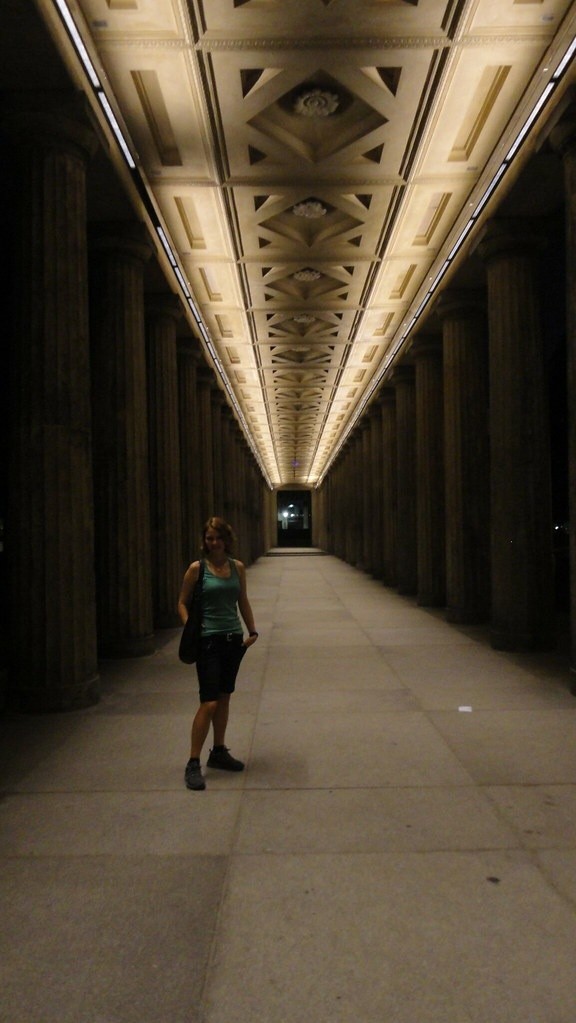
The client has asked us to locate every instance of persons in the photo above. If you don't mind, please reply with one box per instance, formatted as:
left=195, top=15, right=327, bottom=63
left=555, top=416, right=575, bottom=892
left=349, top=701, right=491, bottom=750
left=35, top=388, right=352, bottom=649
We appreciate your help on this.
left=177, top=516, right=258, bottom=791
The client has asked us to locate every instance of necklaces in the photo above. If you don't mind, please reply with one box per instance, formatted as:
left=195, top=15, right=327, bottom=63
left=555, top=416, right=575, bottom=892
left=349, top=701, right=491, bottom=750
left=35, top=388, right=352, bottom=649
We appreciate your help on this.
left=216, top=568, right=221, bottom=571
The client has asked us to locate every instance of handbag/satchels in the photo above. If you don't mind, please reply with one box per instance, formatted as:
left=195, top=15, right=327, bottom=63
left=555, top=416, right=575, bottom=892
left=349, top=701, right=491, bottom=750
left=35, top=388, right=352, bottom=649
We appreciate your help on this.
left=179, top=558, right=205, bottom=664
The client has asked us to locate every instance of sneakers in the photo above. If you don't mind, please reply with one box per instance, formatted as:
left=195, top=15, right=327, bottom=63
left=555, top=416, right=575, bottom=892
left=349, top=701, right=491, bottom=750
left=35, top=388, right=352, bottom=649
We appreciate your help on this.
left=207, top=745, right=245, bottom=772
left=184, top=757, right=205, bottom=790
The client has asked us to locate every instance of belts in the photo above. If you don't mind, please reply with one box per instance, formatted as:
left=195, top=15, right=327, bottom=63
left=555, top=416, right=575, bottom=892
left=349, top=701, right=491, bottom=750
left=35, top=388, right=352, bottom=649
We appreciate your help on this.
left=210, top=633, right=243, bottom=642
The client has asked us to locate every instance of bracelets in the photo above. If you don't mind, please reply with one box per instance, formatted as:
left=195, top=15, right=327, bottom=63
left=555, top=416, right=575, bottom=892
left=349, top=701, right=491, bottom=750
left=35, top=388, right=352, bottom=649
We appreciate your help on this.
left=249, top=632, right=258, bottom=636
